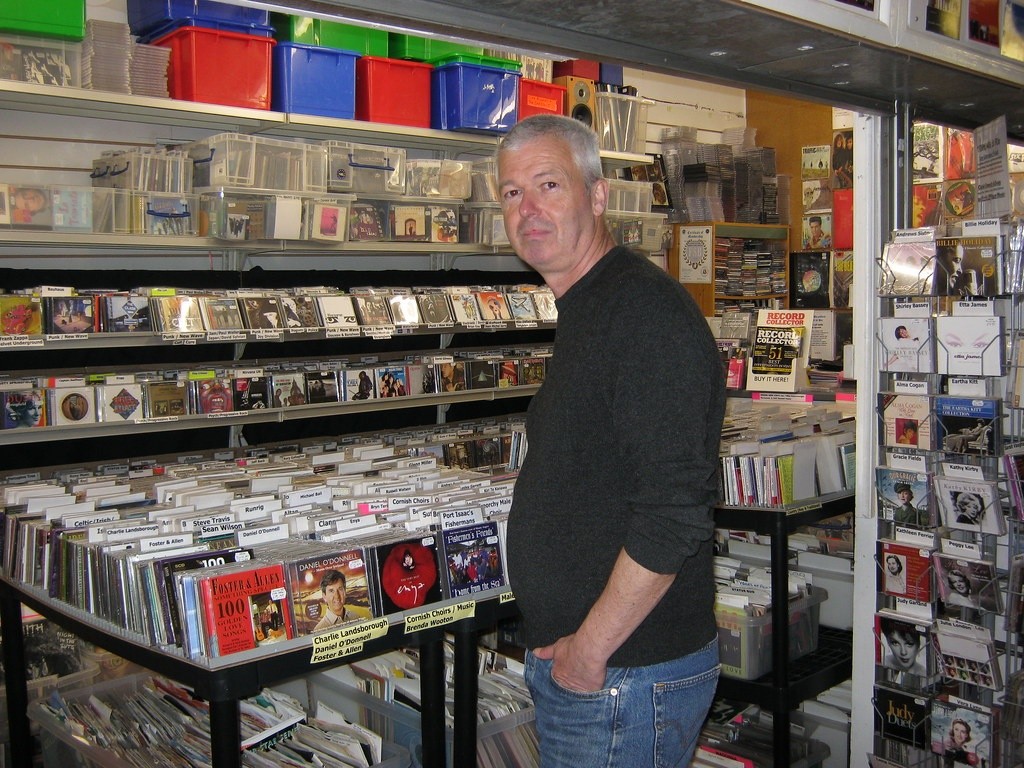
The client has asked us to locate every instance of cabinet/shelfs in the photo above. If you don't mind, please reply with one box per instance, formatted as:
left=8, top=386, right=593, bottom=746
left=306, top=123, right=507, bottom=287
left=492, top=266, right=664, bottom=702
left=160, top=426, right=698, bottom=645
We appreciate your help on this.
left=0, top=574, right=524, bottom=767
left=0, top=78, right=668, bottom=451
left=670, top=222, right=791, bottom=317
left=693, top=490, right=856, bottom=767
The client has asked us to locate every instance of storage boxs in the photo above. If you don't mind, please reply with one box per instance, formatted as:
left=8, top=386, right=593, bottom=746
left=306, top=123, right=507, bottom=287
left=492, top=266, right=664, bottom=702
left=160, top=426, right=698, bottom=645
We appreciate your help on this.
left=693, top=549, right=854, bottom=767
left=0, top=622, right=537, bottom=768
left=0, top=0, right=672, bottom=233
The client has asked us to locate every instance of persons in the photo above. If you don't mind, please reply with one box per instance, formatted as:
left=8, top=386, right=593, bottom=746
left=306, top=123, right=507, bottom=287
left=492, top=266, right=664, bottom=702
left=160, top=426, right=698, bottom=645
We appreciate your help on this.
left=898, top=422, right=917, bottom=444
left=352, top=371, right=372, bottom=400
left=259, top=600, right=279, bottom=639
left=403, top=551, right=415, bottom=571
left=498, top=115, right=720, bottom=768
left=879, top=615, right=926, bottom=688
left=949, top=570, right=984, bottom=609
left=894, top=481, right=923, bottom=528
left=886, top=555, right=906, bottom=594
left=946, top=656, right=993, bottom=687
left=247, top=299, right=279, bottom=328
left=12, top=188, right=52, bottom=213
left=939, top=239, right=974, bottom=295
left=442, top=363, right=466, bottom=393
left=379, top=371, right=407, bottom=398
left=884, top=325, right=921, bottom=370
left=315, top=571, right=359, bottom=630
left=832, top=134, right=853, bottom=187
left=955, top=418, right=985, bottom=452
left=957, top=492, right=981, bottom=524
left=807, top=217, right=831, bottom=248
left=946, top=719, right=972, bottom=751
left=361, top=300, right=388, bottom=324
